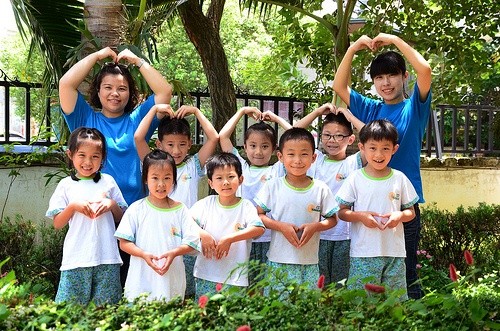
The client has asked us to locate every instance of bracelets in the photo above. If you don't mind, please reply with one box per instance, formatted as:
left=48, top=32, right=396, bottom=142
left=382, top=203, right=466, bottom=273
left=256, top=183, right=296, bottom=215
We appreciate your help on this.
left=136, top=58, right=145, bottom=69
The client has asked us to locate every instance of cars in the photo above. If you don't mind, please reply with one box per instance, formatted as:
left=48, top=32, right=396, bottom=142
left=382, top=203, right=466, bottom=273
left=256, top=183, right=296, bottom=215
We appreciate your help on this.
left=0, top=133, right=26, bottom=141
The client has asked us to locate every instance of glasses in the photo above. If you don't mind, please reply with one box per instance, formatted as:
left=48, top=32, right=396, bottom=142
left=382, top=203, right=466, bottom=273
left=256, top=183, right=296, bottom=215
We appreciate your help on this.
left=320, top=133, right=352, bottom=141
left=100, top=61, right=129, bottom=73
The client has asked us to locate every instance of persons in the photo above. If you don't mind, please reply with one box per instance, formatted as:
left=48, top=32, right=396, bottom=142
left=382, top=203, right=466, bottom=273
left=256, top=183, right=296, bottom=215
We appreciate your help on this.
left=254, top=127, right=339, bottom=297
left=59, top=47, right=172, bottom=295
left=335, top=118, right=419, bottom=303
left=134, top=104, right=219, bottom=299
left=333, top=33, right=431, bottom=299
left=45, top=126, right=128, bottom=305
left=294, top=102, right=365, bottom=292
left=190, top=152, right=267, bottom=296
left=219, top=107, right=293, bottom=296
left=114, top=149, right=201, bottom=304
